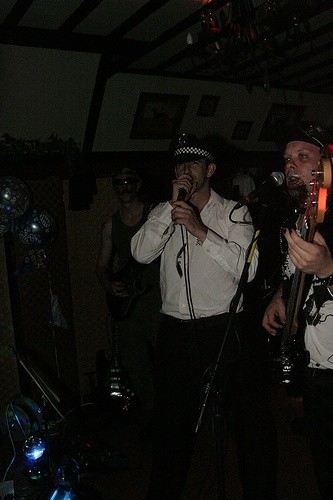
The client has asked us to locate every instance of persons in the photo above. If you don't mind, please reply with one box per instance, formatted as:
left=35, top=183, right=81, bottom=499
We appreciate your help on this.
left=129, top=132, right=276, bottom=500
left=95, top=161, right=163, bottom=414
left=262, top=125, right=333, bottom=500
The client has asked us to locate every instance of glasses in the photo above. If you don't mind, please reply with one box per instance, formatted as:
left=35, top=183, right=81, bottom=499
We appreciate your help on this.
left=112, top=176, right=135, bottom=186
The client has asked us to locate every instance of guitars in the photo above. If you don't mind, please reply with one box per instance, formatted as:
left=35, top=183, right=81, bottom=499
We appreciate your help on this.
left=12, top=342, right=76, bottom=426
left=105, top=256, right=153, bottom=321
left=97, top=322, right=142, bottom=419
left=267, top=158, right=332, bottom=396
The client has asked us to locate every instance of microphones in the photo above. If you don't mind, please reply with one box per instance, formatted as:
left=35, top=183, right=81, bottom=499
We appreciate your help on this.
left=176, top=176, right=192, bottom=207
left=234, top=190, right=258, bottom=209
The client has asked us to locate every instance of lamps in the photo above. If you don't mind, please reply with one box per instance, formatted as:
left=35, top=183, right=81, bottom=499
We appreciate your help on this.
left=184, top=0, right=315, bottom=91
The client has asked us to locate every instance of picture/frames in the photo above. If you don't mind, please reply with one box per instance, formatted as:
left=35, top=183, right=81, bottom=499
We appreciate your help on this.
left=258, top=102, right=306, bottom=141
left=132, top=93, right=189, bottom=140
left=196, top=95, right=219, bottom=118
left=231, top=119, right=254, bottom=140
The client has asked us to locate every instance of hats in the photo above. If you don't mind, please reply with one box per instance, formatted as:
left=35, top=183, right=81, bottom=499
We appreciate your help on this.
left=168, top=133, right=209, bottom=168
left=284, top=120, right=333, bottom=149
